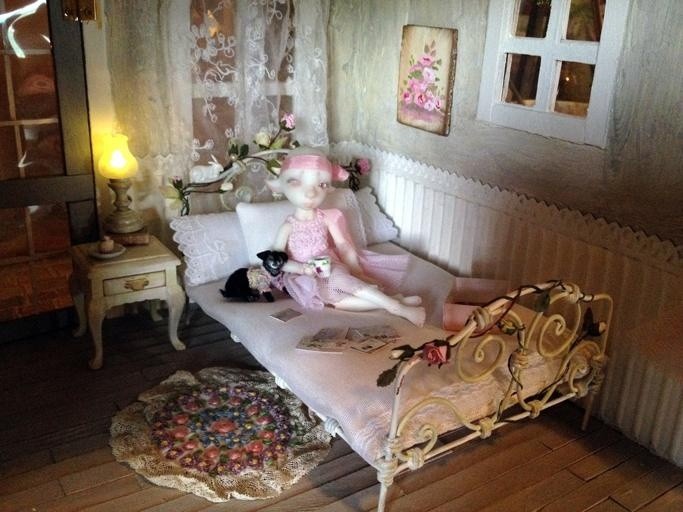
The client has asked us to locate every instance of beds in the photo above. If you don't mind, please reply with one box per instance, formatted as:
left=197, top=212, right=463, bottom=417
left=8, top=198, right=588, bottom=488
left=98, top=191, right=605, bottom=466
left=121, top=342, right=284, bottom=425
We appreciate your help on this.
left=170, top=146, right=616, bottom=511
left=70, top=234, right=187, bottom=370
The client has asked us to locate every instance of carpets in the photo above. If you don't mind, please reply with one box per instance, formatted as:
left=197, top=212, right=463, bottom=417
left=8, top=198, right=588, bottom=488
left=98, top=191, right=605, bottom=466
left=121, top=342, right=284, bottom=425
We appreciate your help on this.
left=106, top=363, right=335, bottom=506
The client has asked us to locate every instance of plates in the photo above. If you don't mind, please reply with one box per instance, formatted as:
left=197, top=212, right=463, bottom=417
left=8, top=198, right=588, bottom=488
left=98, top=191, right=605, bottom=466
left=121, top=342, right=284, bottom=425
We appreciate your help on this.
left=88, top=242, right=125, bottom=259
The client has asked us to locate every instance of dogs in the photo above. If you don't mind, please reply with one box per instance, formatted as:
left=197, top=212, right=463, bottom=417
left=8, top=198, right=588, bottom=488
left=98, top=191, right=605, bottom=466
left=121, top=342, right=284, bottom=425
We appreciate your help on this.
left=219, top=250, right=291, bottom=302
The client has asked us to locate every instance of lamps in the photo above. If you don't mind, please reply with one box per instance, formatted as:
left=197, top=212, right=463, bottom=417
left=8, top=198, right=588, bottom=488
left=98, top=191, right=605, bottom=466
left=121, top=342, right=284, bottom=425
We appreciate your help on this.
left=96, top=132, right=146, bottom=234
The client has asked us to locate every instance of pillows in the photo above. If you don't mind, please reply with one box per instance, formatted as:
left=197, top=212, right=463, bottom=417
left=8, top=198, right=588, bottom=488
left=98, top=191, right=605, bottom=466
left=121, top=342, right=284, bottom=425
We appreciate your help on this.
left=233, top=187, right=369, bottom=270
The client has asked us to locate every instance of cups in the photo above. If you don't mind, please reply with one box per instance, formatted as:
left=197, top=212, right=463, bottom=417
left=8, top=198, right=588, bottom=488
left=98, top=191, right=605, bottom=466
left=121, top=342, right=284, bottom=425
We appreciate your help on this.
left=308, top=255, right=331, bottom=279
